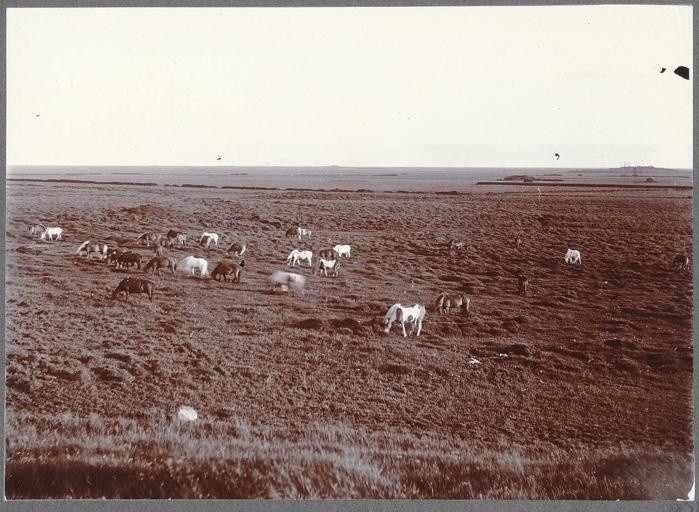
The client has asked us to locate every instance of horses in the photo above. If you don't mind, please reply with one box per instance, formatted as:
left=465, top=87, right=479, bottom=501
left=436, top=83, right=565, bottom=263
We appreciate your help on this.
left=76, top=229, right=246, bottom=285
left=382, top=303, right=426, bottom=338
left=269, top=271, right=305, bottom=294
left=284, top=227, right=353, bottom=277
left=672, top=254, right=689, bottom=269
left=563, top=249, right=583, bottom=265
left=40, top=226, right=65, bottom=242
left=27, top=222, right=46, bottom=236
left=518, top=275, right=528, bottom=293
left=437, top=294, right=472, bottom=315
left=111, top=278, right=155, bottom=302
